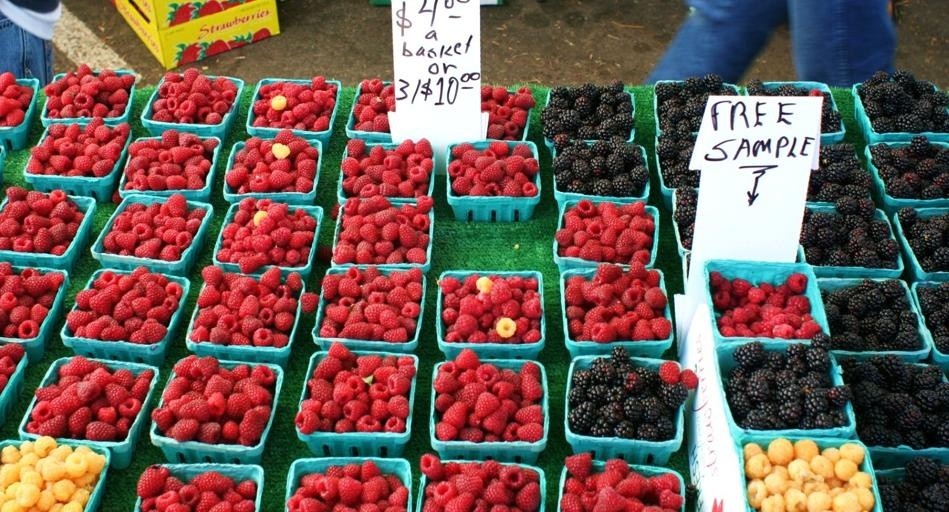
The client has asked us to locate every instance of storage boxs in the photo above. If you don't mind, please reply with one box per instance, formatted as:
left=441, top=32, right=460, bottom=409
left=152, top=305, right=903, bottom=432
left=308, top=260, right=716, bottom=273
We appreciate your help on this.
left=112, top=0, right=283, bottom=70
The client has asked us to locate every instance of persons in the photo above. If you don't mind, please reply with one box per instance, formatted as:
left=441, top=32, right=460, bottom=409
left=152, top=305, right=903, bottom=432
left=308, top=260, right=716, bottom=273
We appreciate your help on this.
left=0, top=0, right=63, bottom=89
left=645, top=1, right=901, bottom=89
